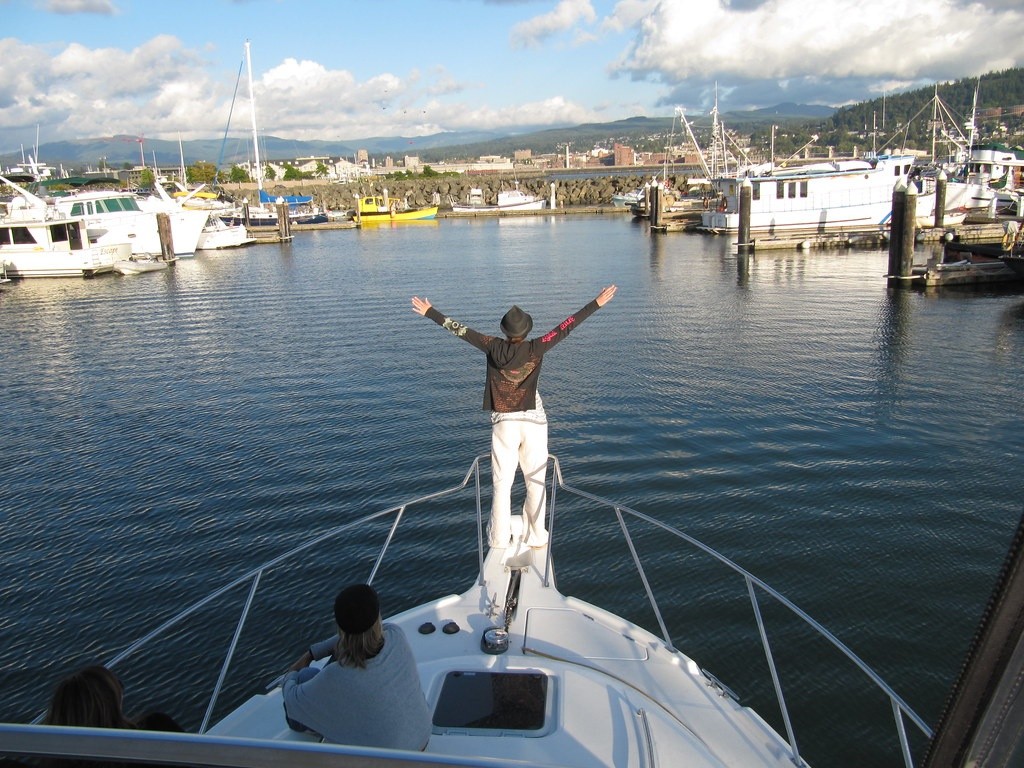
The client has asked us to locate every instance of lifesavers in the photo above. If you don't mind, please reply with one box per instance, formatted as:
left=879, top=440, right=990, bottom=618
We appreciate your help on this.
left=722, top=199, right=728, bottom=209
left=702, top=197, right=709, bottom=208
left=1001, top=232, right=1015, bottom=252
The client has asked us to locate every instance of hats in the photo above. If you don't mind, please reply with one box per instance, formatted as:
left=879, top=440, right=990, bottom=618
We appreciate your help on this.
left=500, top=305, right=533, bottom=337
left=334, top=583, right=380, bottom=635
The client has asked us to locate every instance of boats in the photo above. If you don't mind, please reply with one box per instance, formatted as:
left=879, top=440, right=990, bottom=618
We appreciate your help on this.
left=220, top=191, right=328, bottom=228
left=447, top=182, right=547, bottom=211
left=24, top=175, right=214, bottom=260
left=694, top=81, right=1024, bottom=233
left=0, top=174, right=133, bottom=279
left=112, top=254, right=168, bottom=275
left=354, top=196, right=439, bottom=221
left=129, top=181, right=257, bottom=252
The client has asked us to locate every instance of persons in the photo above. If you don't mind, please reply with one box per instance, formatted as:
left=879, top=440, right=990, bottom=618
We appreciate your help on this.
left=40, top=666, right=185, bottom=768
left=411, top=285, right=618, bottom=548
left=283, top=585, right=432, bottom=752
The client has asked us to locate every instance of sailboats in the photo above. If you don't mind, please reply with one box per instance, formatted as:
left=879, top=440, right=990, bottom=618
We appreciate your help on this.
left=192, top=37, right=328, bottom=227
left=610, top=106, right=684, bottom=206
